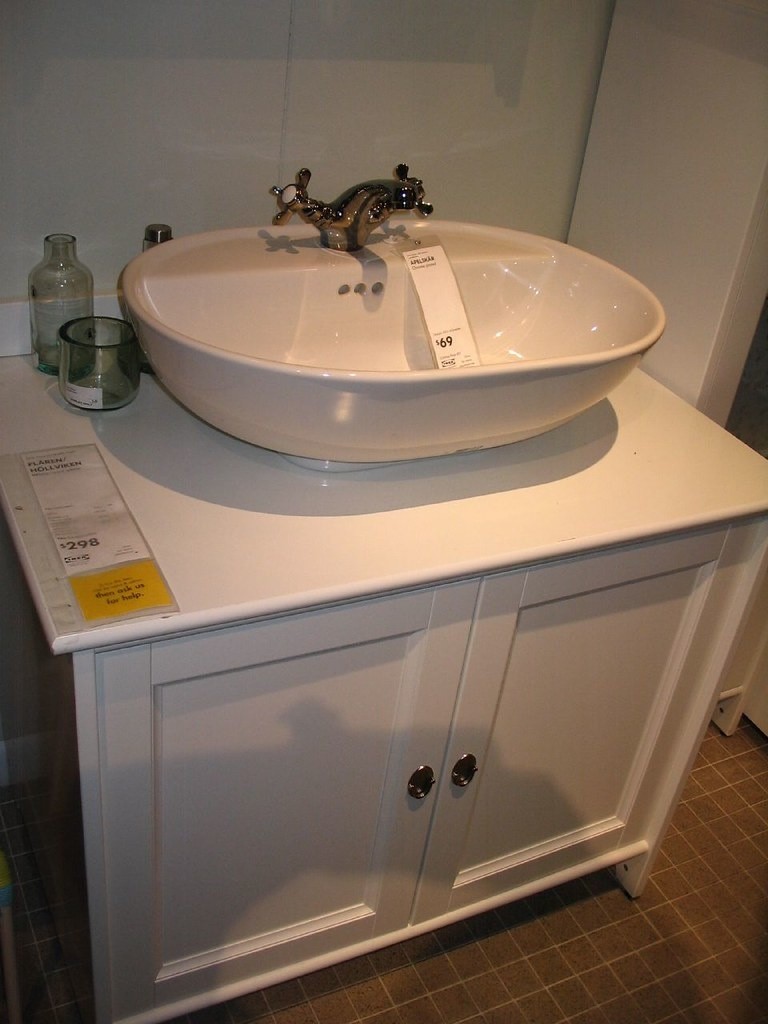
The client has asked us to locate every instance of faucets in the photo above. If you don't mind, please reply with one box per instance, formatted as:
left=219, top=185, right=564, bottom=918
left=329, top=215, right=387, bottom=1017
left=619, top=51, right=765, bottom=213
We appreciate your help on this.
left=271, top=161, right=434, bottom=254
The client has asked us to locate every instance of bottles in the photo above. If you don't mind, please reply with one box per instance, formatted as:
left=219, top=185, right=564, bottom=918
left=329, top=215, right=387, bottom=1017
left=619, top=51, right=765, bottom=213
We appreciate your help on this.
left=27, top=234, right=93, bottom=375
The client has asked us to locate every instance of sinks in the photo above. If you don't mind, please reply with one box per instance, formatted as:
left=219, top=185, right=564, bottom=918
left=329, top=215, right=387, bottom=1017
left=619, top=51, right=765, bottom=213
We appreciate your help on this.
left=116, top=216, right=668, bottom=464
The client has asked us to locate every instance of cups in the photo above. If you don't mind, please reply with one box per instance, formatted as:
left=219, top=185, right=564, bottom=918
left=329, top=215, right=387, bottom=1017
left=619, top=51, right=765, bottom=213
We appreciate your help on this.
left=57, top=315, right=141, bottom=410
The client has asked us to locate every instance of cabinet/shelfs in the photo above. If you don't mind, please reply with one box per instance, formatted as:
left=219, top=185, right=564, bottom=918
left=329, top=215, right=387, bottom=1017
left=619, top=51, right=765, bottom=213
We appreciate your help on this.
left=0, top=511, right=768, bottom=1024
